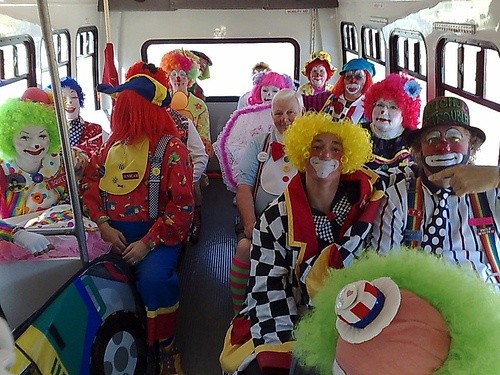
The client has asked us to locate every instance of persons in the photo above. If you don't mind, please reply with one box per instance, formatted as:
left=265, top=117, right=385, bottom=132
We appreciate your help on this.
left=368, top=98, right=500, bottom=289
left=231, top=53, right=421, bottom=319
left=288, top=249, right=500, bottom=375
left=247, top=112, right=421, bottom=375
left=84, top=76, right=195, bottom=375
left=46, top=50, right=212, bottom=238
left=0, top=88, right=111, bottom=262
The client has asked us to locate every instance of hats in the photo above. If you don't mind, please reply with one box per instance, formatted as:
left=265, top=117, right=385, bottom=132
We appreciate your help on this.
left=402, top=96, right=486, bottom=151
left=340, top=58, right=376, bottom=78
left=300, top=51, right=337, bottom=76
left=191, top=49, right=212, bottom=67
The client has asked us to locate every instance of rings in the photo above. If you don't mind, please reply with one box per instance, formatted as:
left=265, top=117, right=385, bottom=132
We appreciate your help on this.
left=133, top=261, right=136, bottom=265
